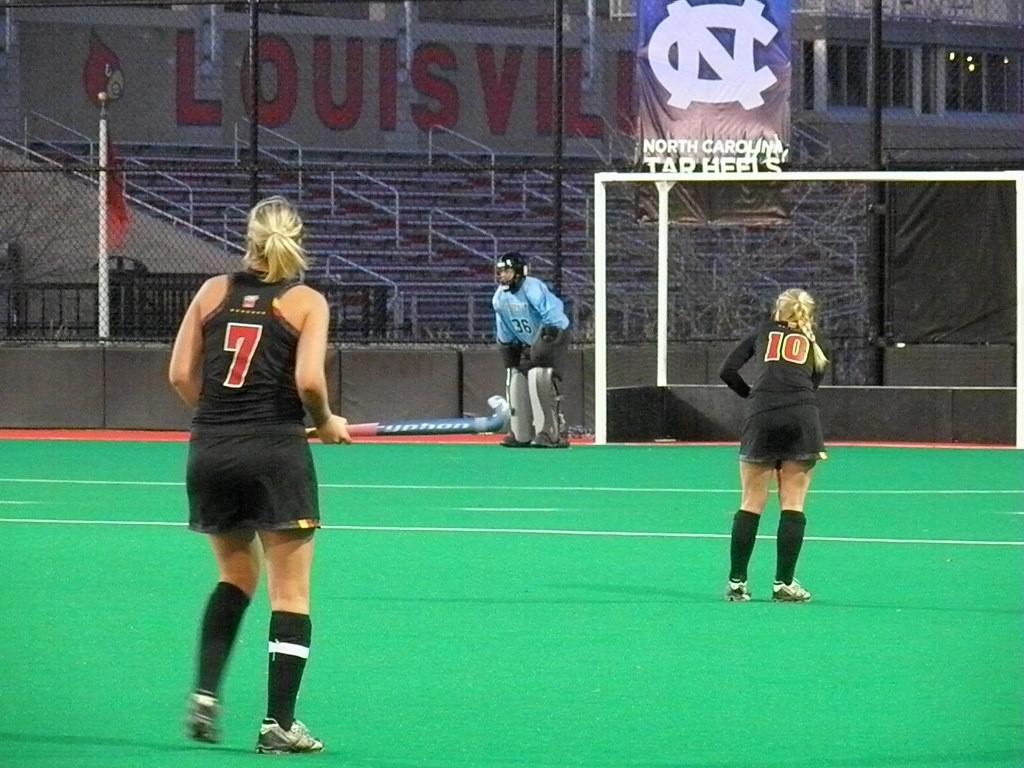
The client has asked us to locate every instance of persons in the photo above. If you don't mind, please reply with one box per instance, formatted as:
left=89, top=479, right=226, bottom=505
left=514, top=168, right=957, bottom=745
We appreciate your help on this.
left=491, top=249, right=577, bottom=449
left=714, top=286, right=831, bottom=603
left=168, top=192, right=332, bottom=757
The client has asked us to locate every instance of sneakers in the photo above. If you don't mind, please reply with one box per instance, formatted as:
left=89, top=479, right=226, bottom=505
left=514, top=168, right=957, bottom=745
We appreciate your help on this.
left=531, top=430, right=560, bottom=448
left=184, top=693, right=223, bottom=745
left=771, top=576, right=811, bottom=603
left=255, top=716, right=325, bottom=756
left=500, top=431, right=531, bottom=448
left=723, top=580, right=751, bottom=603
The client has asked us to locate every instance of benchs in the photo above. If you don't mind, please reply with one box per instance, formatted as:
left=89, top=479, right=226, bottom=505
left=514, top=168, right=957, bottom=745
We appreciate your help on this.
left=27, top=142, right=869, bottom=342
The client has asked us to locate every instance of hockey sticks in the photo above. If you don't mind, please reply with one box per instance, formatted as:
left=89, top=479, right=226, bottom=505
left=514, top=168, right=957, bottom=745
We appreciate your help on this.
left=305, top=396, right=513, bottom=440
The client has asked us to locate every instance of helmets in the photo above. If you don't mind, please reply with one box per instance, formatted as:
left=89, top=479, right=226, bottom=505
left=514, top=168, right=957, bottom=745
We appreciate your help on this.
left=496, top=252, right=528, bottom=294
